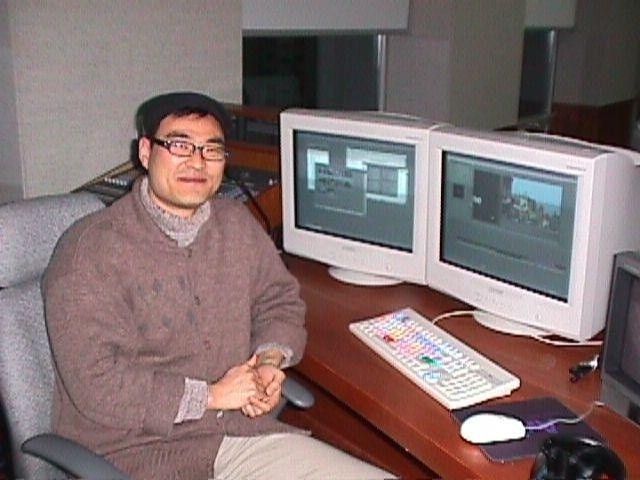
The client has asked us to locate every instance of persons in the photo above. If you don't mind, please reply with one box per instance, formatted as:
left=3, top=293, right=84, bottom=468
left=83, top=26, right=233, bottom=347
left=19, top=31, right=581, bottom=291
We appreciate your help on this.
left=40, top=94, right=398, bottom=479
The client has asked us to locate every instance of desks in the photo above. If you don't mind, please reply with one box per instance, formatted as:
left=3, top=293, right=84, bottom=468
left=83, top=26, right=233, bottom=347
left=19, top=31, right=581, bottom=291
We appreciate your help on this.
left=271, top=253, right=639, bottom=480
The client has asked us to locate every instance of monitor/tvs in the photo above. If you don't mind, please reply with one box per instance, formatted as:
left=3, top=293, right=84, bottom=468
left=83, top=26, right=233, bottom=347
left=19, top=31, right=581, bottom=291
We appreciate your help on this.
left=598, top=249, right=640, bottom=428
left=279, top=105, right=455, bottom=288
left=427, top=121, right=640, bottom=338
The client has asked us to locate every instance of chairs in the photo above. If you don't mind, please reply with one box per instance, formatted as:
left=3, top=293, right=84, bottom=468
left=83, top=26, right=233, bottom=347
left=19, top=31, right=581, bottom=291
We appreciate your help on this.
left=0, top=190, right=314, bottom=480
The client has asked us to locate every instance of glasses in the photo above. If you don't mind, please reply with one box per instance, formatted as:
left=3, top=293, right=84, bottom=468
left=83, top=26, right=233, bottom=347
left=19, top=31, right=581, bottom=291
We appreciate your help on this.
left=147, top=135, right=229, bottom=162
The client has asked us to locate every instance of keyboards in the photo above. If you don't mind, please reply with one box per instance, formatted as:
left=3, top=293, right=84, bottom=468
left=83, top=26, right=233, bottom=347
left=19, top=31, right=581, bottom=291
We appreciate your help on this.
left=348, top=303, right=522, bottom=409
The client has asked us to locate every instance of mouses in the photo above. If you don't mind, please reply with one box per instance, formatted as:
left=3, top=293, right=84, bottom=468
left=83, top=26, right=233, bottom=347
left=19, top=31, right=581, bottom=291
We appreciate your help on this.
left=459, top=412, right=526, bottom=445
